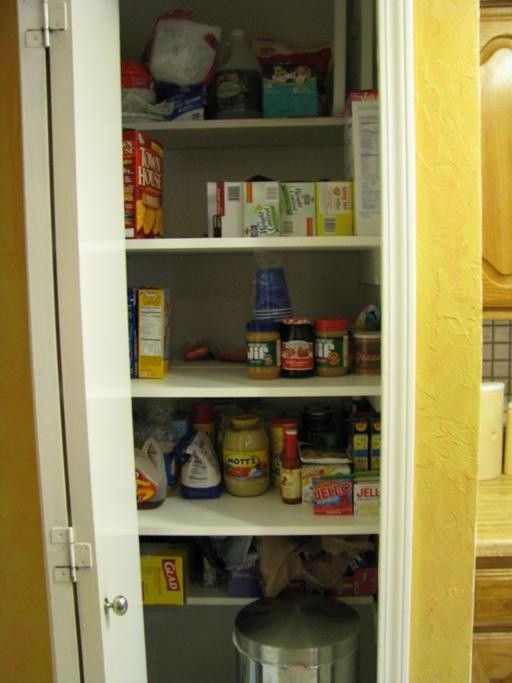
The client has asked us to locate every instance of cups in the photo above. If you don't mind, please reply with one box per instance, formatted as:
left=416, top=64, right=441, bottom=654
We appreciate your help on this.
left=251, top=267, right=294, bottom=320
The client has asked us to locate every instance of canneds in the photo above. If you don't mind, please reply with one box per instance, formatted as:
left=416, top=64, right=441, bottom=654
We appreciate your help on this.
left=220, top=415, right=271, bottom=497
left=280, top=317, right=315, bottom=378
left=246, top=320, right=280, bottom=380
left=313, top=318, right=351, bottom=377
left=350, top=331, right=380, bottom=375
left=299, top=404, right=336, bottom=450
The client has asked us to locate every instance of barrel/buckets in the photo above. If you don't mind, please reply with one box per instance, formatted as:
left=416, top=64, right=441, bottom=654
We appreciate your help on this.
left=231, top=594, right=362, bottom=679
left=135, top=436, right=168, bottom=510
left=213, top=25, right=263, bottom=117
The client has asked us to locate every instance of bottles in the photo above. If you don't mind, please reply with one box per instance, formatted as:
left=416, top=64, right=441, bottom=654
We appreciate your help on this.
left=279, top=423, right=302, bottom=505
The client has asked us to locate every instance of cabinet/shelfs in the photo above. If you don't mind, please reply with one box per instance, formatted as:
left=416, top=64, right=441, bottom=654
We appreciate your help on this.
left=118, top=0, right=413, bottom=683
